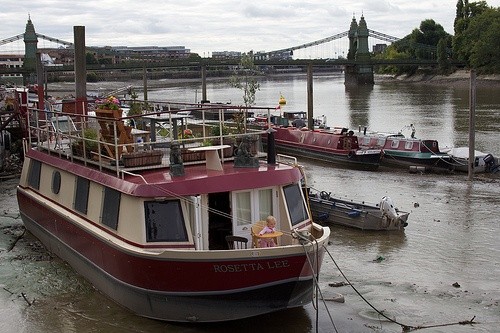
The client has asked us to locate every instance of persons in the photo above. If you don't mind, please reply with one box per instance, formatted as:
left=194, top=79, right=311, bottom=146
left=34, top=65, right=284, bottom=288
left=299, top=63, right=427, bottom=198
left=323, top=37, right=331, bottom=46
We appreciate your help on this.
left=28, top=79, right=38, bottom=84
left=258, top=216, right=278, bottom=248
left=320, top=114, right=326, bottom=129
left=130, top=104, right=162, bottom=128
left=407, top=124, right=415, bottom=139
left=46, top=96, right=52, bottom=120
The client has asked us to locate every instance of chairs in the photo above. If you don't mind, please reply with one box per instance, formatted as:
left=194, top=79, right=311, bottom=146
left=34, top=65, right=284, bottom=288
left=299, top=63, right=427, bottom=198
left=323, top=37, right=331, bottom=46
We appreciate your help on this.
left=50, top=116, right=82, bottom=152
left=250, top=221, right=283, bottom=247
left=225, top=235, right=249, bottom=249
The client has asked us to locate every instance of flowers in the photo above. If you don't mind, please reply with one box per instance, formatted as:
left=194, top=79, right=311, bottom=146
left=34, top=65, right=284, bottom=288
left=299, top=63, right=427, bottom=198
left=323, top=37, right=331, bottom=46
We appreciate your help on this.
left=180, top=128, right=196, bottom=143
left=93, top=97, right=121, bottom=110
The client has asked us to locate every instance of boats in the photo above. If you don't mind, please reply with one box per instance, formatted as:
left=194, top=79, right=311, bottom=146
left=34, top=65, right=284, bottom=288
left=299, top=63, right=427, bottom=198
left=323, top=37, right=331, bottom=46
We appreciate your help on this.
left=16, top=93, right=332, bottom=324
left=260, top=114, right=385, bottom=170
left=444, top=147, right=500, bottom=174
left=343, top=123, right=453, bottom=174
left=306, top=190, right=410, bottom=231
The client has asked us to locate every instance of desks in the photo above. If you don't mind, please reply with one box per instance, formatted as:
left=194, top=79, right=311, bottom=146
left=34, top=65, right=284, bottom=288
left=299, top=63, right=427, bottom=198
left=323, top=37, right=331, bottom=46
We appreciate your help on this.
left=131, top=127, right=152, bottom=150
left=142, top=114, right=188, bottom=142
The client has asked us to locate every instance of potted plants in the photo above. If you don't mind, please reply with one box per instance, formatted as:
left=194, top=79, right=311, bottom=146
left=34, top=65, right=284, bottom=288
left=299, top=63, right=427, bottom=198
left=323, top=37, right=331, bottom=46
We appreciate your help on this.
left=210, top=125, right=228, bottom=158
left=228, top=55, right=262, bottom=167
left=70, top=127, right=102, bottom=159
